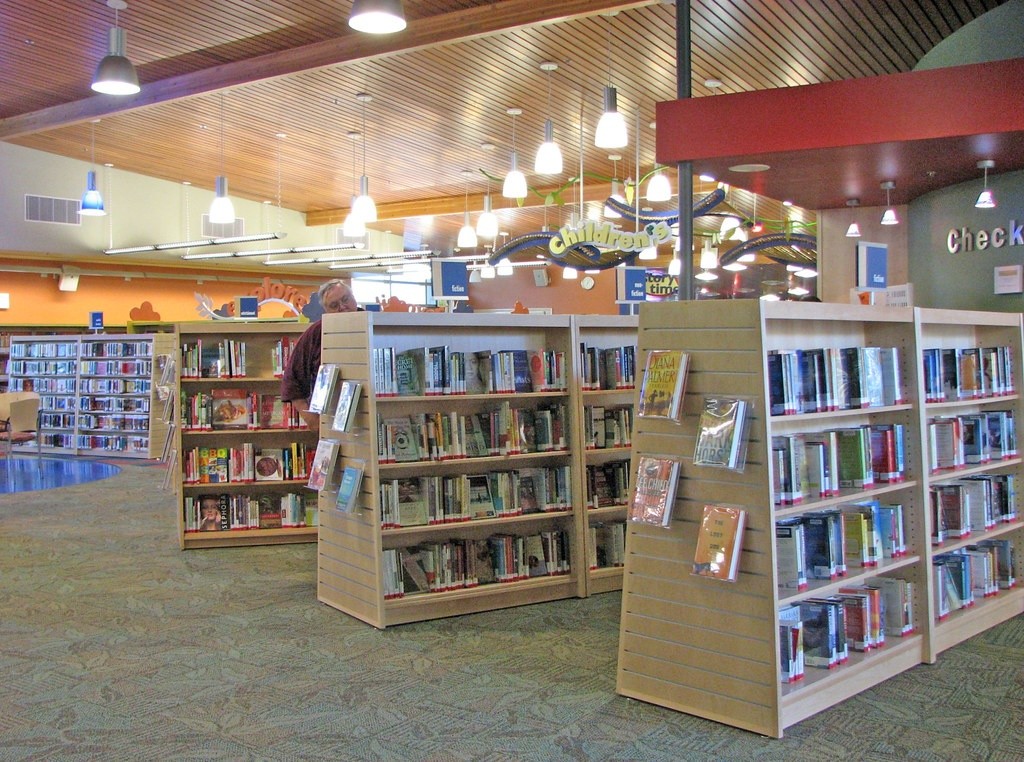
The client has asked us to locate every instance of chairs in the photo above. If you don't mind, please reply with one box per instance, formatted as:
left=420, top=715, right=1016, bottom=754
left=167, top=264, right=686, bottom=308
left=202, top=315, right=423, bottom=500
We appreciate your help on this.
left=0, top=391, right=44, bottom=477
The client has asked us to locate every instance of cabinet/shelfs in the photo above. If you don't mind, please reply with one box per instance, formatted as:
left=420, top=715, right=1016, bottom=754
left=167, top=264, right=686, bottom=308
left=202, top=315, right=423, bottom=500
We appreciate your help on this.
left=1, top=302, right=1022, bottom=739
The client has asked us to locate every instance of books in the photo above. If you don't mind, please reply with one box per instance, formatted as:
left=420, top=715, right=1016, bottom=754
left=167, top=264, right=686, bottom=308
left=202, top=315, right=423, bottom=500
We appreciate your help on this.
left=335, top=468, right=361, bottom=513
left=638, top=350, right=689, bottom=421
left=692, top=398, right=748, bottom=469
left=307, top=440, right=340, bottom=491
left=309, top=364, right=362, bottom=434
left=767, top=347, right=915, bottom=686
left=632, top=457, right=681, bottom=527
left=9, top=342, right=152, bottom=450
left=373, top=342, right=637, bottom=600
left=923, top=345, right=1017, bottom=622
left=692, top=505, right=747, bottom=581
left=159, top=338, right=319, bottom=532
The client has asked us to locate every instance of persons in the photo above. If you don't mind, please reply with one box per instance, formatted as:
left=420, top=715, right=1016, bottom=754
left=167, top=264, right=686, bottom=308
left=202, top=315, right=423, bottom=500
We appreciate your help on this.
left=200, top=498, right=222, bottom=531
left=281, top=279, right=365, bottom=434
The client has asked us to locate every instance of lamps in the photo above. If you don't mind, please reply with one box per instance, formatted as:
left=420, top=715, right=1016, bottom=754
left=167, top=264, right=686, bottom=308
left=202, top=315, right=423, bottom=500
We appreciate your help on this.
left=881, top=182, right=899, bottom=224
left=534, top=61, right=564, bottom=177
left=593, top=11, right=629, bottom=149
left=90, top=0, right=143, bottom=96
left=209, top=93, right=235, bottom=222
left=345, top=1, right=405, bottom=34
left=457, top=107, right=529, bottom=248
left=977, top=158, right=1000, bottom=209
left=75, top=120, right=109, bottom=216
left=845, top=199, right=865, bottom=238
left=559, top=154, right=818, bottom=301
left=467, top=231, right=513, bottom=283
left=344, top=94, right=378, bottom=236
